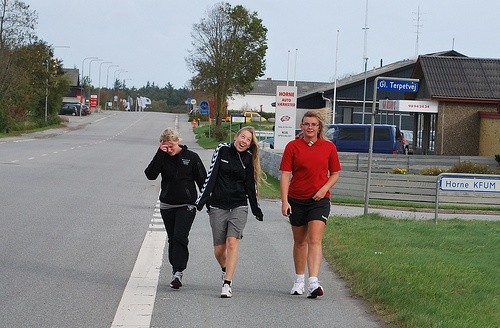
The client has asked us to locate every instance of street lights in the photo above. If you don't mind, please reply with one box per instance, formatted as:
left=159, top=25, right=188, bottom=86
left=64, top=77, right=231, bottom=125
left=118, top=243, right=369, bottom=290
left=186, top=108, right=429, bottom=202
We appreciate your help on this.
left=113, top=69, right=128, bottom=110
left=98, top=61, right=112, bottom=106
left=44, top=45, right=70, bottom=122
left=79, top=56, right=98, bottom=118
left=88, top=58, right=105, bottom=101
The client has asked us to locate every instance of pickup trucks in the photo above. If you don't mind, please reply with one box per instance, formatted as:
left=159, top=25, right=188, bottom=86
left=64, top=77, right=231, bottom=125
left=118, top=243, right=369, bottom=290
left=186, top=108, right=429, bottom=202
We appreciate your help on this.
left=227, top=112, right=267, bottom=122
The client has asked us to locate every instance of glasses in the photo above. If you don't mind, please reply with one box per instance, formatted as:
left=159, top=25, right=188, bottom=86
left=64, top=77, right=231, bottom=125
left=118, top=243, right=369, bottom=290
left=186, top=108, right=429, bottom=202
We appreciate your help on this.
left=300, top=123, right=319, bottom=127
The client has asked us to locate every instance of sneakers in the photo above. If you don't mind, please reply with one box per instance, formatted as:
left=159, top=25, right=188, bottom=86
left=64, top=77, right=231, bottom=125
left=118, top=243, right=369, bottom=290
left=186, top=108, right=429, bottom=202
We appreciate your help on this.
left=221, top=272, right=232, bottom=298
left=290, top=281, right=304, bottom=295
left=306, top=281, right=324, bottom=299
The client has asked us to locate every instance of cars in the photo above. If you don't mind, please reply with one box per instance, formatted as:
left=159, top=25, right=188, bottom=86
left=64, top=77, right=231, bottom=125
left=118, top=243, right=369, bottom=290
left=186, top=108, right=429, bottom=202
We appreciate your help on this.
left=258, top=129, right=302, bottom=149
left=404, top=142, right=409, bottom=155
left=58, top=102, right=89, bottom=116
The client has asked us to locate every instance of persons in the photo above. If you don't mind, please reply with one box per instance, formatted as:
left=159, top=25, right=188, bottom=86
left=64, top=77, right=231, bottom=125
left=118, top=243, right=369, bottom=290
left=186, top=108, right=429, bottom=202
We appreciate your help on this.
left=194, top=125, right=270, bottom=298
left=145, top=128, right=207, bottom=289
left=279, top=111, right=341, bottom=298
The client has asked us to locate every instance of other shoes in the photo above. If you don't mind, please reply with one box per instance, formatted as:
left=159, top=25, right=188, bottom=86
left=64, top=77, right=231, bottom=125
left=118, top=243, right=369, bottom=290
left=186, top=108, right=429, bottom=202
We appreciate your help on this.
left=170, top=270, right=183, bottom=289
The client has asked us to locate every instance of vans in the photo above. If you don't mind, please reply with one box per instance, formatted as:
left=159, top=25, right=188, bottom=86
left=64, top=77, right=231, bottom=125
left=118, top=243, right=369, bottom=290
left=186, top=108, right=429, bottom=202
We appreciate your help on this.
left=322, top=124, right=406, bottom=153
